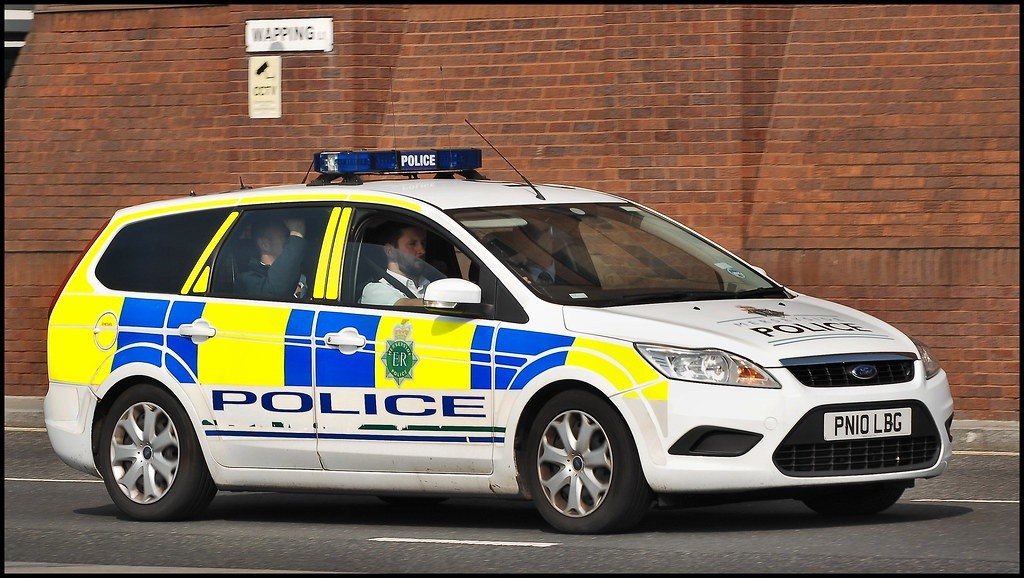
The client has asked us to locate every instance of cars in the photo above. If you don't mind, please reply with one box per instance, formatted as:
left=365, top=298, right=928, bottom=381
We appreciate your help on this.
left=45, top=148, right=955, bottom=535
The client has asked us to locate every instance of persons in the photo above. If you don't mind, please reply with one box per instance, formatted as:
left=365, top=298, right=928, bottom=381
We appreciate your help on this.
left=361, top=218, right=531, bottom=306
left=229, top=209, right=310, bottom=299
left=512, top=211, right=599, bottom=297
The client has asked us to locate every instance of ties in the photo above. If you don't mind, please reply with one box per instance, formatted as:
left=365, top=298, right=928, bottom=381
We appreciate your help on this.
left=540, top=271, right=550, bottom=284
